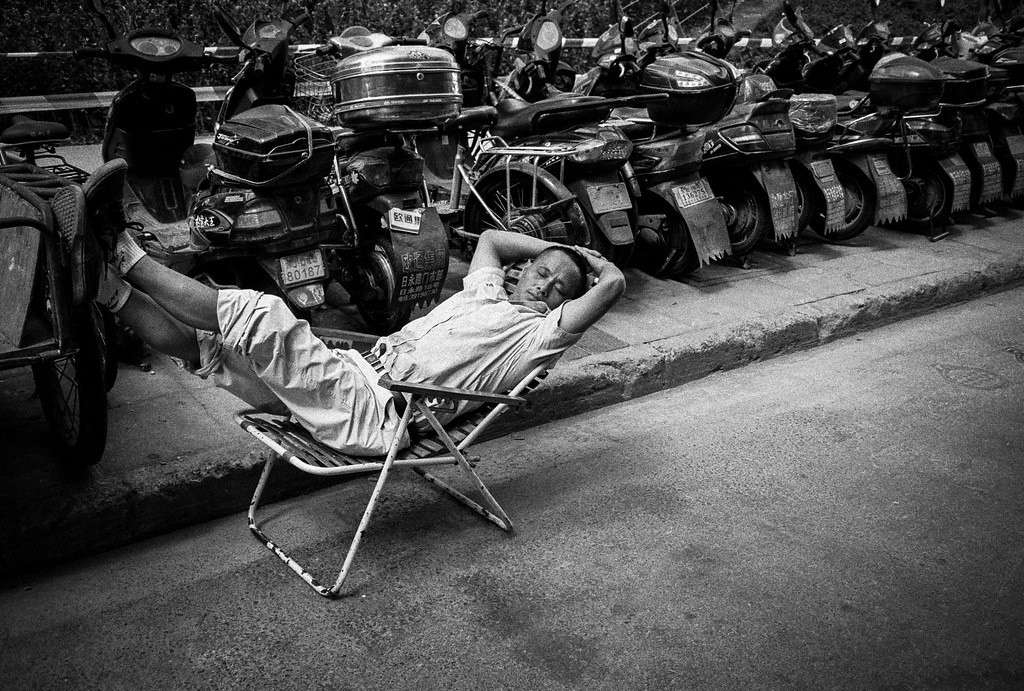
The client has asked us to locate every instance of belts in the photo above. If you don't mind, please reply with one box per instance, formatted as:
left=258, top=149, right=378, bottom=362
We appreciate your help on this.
left=361, top=350, right=420, bottom=446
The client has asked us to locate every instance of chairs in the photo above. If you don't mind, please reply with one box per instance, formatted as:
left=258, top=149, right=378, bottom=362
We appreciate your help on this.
left=233, top=328, right=563, bottom=598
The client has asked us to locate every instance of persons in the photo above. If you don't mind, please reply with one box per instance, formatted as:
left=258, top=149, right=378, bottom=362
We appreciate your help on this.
left=50, top=157, right=626, bottom=455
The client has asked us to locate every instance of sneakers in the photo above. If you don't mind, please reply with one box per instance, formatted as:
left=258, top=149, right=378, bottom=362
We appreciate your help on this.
left=50, top=184, right=100, bottom=303
left=83, top=158, right=145, bottom=280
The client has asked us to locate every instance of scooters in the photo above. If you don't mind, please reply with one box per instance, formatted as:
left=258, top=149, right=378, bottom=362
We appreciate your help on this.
left=0, top=0, right=1024, bottom=471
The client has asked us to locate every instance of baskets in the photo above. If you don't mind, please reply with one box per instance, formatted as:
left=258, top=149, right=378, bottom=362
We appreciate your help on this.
left=465, top=44, right=492, bottom=103
left=288, top=53, right=342, bottom=123
